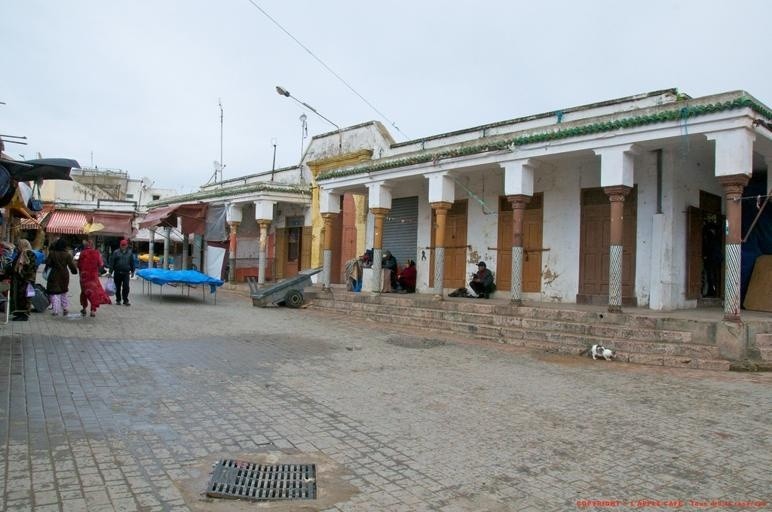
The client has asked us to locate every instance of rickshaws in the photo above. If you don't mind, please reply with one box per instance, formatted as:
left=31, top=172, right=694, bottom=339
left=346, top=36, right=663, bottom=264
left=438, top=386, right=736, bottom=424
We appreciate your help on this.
left=247, top=264, right=323, bottom=309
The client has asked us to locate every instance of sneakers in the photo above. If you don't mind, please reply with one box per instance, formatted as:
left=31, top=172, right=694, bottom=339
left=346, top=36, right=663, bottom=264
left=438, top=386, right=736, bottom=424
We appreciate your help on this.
left=52, top=309, right=68, bottom=316
left=476, top=293, right=490, bottom=299
left=12, top=312, right=29, bottom=321
left=81, top=309, right=96, bottom=316
left=116, top=299, right=131, bottom=306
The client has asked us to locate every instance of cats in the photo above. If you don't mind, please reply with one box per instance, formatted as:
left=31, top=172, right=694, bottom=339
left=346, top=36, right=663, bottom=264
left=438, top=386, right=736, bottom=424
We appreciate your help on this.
left=579, top=344, right=617, bottom=361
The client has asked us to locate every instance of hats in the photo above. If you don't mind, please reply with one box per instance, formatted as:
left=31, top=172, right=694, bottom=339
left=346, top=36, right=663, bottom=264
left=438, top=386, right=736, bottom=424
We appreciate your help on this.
left=476, top=261, right=486, bottom=266
left=120, top=240, right=128, bottom=245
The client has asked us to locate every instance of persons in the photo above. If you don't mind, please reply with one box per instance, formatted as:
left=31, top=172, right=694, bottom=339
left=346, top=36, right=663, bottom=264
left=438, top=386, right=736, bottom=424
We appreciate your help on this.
left=44, top=239, right=77, bottom=315
left=108, top=241, right=135, bottom=305
left=12, top=239, right=37, bottom=321
left=397, top=260, right=416, bottom=293
left=382, top=251, right=396, bottom=286
left=78, top=240, right=111, bottom=317
left=470, top=262, right=495, bottom=298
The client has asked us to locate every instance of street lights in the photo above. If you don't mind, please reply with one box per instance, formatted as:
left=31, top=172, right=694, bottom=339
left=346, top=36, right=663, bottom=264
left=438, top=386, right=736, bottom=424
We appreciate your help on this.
left=276, top=85, right=342, bottom=157
